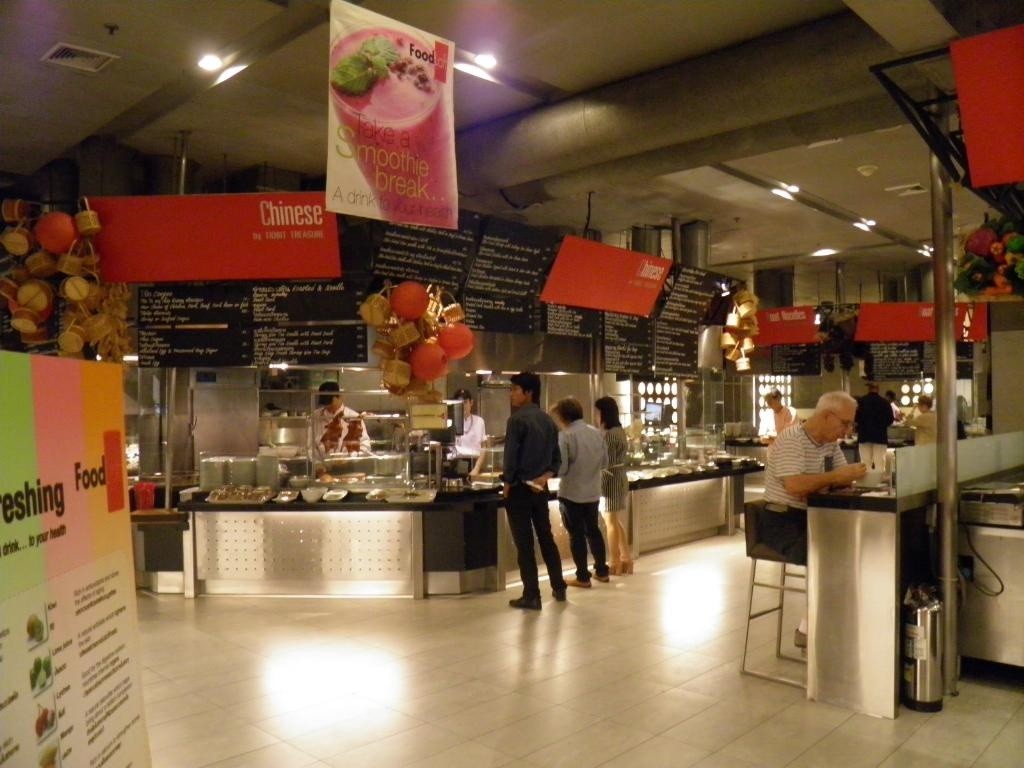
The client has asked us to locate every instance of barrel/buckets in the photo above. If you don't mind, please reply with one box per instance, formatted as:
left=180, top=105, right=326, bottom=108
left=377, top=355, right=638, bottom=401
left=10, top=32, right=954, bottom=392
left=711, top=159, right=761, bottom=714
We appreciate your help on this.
left=133, top=481, right=156, bottom=510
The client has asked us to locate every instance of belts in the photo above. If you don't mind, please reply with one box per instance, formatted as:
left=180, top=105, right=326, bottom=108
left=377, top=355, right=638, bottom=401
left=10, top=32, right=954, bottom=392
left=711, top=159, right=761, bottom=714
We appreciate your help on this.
left=764, top=502, right=789, bottom=514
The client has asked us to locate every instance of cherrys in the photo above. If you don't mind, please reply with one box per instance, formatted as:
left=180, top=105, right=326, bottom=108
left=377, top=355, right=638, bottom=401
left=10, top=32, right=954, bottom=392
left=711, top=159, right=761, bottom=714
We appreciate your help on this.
left=35, top=704, right=55, bottom=737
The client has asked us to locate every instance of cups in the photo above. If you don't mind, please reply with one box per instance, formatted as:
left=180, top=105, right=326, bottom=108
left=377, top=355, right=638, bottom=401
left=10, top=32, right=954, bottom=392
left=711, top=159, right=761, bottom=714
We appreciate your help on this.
left=330, top=17, right=458, bottom=232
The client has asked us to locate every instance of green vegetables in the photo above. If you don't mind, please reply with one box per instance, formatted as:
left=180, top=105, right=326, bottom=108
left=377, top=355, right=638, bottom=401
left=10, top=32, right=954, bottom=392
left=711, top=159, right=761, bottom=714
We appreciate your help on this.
left=953, top=214, right=1024, bottom=298
left=331, top=37, right=401, bottom=93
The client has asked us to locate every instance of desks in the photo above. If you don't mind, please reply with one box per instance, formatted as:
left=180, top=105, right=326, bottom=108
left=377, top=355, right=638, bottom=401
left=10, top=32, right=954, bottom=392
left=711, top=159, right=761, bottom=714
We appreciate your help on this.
left=802, top=429, right=1024, bottom=720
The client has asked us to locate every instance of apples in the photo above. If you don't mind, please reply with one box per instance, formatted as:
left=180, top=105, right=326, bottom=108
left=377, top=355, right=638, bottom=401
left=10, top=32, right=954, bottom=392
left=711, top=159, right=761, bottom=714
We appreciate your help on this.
left=26, top=614, right=44, bottom=641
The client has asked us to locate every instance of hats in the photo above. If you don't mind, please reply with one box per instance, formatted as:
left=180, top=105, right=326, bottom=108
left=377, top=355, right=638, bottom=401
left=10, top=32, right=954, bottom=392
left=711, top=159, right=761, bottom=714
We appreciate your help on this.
left=317, top=381, right=341, bottom=405
left=452, top=388, right=471, bottom=400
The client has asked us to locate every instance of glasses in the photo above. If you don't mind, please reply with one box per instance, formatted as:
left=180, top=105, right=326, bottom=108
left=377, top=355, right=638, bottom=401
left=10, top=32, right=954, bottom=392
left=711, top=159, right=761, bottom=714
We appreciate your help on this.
left=830, top=410, right=859, bottom=430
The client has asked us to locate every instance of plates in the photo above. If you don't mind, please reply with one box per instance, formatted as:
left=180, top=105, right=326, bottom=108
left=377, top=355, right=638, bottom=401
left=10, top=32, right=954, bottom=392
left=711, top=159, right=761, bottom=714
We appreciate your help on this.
left=734, top=435, right=751, bottom=444
left=270, top=473, right=390, bottom=503
left=625, top=456, right=723, bottom=486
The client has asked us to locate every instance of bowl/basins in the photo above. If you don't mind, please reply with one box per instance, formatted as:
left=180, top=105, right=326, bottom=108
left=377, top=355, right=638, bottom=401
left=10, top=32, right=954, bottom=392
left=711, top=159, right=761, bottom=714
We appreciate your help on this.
left=856, top=469, right=882, bottom=487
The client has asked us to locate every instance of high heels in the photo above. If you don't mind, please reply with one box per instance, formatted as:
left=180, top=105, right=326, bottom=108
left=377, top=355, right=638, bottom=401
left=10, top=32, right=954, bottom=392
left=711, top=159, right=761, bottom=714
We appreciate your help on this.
left=608, top=559, right=634, bottom=576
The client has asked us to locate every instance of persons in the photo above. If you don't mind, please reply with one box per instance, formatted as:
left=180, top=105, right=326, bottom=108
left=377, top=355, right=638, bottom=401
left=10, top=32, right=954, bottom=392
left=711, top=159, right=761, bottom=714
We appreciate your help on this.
left=497, top=370, right=568, bottom=609
left=591, top=397, right=635, bottom=576
left=307, top=381, right=372, bottom=463
left=854, top=382, right=894, bottom=472
left=904, top=395, right=937, bottom=445
left=550, top=395, right=610, bottom=587
left=444, top=388, right=488, bottom=476
left=764, top=387, right=799, bottom=436
left=761, top=390, right=870, bottom=646
left=885, top=389, right=900, bottom=422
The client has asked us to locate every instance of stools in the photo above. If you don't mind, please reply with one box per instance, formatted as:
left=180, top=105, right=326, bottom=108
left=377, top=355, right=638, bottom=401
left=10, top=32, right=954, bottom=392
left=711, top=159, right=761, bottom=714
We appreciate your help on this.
left=739, top=500, right=808, bottom=692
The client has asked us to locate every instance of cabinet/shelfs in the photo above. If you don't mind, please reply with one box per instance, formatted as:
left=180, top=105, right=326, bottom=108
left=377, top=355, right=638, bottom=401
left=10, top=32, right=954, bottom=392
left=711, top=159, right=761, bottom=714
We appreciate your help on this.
left=257, top=362, right=412, bottom=487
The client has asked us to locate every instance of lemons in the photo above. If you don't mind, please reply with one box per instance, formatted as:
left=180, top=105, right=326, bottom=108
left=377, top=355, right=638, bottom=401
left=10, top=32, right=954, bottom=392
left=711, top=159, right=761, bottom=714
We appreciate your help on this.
left=29, top=655, right=52, bottom=691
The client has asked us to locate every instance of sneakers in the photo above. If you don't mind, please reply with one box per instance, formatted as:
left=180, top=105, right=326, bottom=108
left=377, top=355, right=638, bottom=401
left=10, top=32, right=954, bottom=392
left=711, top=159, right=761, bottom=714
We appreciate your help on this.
left=794, top=628, right=809, bottom=648
left=552, top=587, right=567, bottom=602
left=509, top=595, right=542, bottom=610
left=564, top=575, right=592, bottom=588
left=590, top=568, right=609, bottom=583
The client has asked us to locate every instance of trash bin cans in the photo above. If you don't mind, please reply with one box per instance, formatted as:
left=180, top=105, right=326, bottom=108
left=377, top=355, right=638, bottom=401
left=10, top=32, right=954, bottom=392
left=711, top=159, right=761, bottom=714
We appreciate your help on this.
left=906, top=586, right=948, bottom=712
